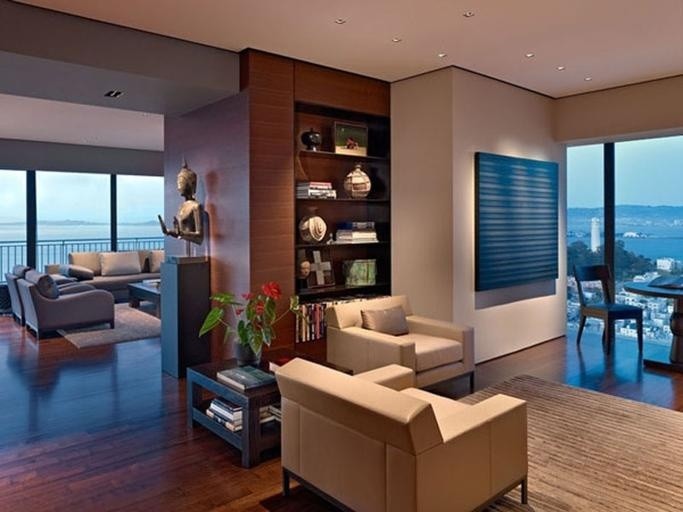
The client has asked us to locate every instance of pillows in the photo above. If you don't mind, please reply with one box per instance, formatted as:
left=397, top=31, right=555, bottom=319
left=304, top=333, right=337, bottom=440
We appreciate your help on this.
left=58, top=265, right=95, bottom=281
left=359, top=303, right=409, bottom=338
left=148, top=250, right=165, bottom=273
left=98, top=251, right=142, bottom=276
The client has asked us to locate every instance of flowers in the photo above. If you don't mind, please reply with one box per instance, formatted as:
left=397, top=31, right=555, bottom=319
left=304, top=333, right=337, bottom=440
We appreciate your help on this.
left=200, top=282, right=299, bottom=354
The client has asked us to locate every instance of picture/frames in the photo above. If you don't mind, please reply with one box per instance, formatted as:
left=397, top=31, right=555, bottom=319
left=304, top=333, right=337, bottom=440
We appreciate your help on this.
left=332, top=120, right=369, bottom=157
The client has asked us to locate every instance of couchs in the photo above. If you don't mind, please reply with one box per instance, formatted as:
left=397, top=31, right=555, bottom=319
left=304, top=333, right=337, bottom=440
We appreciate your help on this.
left=67, top=250, right=164, bottom=299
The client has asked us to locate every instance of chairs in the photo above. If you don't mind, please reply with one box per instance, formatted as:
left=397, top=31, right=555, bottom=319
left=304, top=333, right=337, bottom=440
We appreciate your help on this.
left=573, top=263, right=645, bottom=355
left=17, top=279, right=116, bottom=338
left=4, top=264, right=83, bottom=327
left=321, top=293, right=475, bottom=398
left=272, top=357, right=529, bottom=512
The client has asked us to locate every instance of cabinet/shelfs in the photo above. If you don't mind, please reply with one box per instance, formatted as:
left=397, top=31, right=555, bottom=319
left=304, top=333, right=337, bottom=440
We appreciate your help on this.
left=292, top=97, right=391, bottom=345
left=186, top=349, right=353, bottom=469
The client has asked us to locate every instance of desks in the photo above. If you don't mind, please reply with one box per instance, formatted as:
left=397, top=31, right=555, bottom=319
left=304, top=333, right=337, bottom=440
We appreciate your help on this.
left=624, top=273, right=683, bottom=374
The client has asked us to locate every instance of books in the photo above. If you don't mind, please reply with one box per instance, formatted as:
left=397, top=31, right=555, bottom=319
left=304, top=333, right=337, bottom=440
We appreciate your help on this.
left=335, top=221, right=377, bottom=241
left=304, top=247, right=336, bottom=289
left=205, top=396, right=280, bottom=432
left=294, top=293, right=383, bottom=343
left=295, top=181, right=337, bottom=200
left=215, top=365, right=275, bottom=390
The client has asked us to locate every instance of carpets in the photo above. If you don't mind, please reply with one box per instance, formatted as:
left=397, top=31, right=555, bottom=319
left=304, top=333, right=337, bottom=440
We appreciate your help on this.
left=456, top=374, right=683, bottom=512
left=56, top=300, right=161, bottom=349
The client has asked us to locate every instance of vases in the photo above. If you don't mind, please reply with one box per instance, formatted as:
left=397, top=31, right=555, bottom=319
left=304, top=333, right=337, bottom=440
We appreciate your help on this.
left=344, top=161, right=372, bottom=200
left=232, top=338, right=264, bottom=371
left=298, top=205, right=327, bottom=244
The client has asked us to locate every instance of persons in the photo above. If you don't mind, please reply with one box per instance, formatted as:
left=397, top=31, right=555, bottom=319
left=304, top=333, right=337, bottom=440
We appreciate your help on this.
left=157, top=157, right=203, bottom=245
left=342, top=135, right=359, bottom=150
left=298, top=261, right=310, bottom=286
